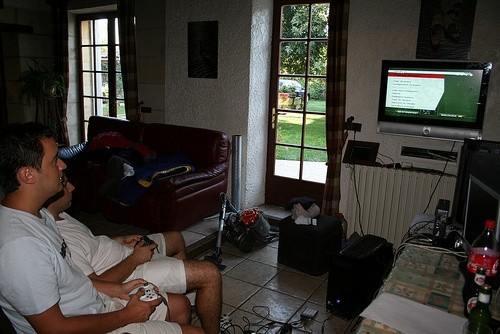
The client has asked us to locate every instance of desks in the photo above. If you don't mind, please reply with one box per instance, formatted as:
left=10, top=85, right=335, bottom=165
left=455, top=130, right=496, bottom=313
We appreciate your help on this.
left=352, top=213, right=500, bottom=334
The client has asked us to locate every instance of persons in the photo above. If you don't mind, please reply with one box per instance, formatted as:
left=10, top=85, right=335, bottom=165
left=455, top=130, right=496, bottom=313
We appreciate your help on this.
left=0, top=122, right=207, bottom=334
left=44, top=169, right=222, bottom=334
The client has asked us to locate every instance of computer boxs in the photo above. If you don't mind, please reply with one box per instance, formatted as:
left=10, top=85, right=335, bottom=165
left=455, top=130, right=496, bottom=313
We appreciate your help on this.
left=326, top=236, right=393, bottom=322
left=450, top=139, right=500, bottom=230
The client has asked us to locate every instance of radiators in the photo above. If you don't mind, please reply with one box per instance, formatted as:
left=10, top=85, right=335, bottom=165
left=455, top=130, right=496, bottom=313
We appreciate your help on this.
left=345, top=164, right=457, bottom=254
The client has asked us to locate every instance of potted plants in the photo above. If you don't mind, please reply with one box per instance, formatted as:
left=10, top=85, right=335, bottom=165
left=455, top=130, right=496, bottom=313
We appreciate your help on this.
left=15, top=57, right=66, bottom=108
left=47, top=117, right=58, bottom=142
left=278, top=85, right=291, bottom=98
left=288, top=86, right=297, bottom=97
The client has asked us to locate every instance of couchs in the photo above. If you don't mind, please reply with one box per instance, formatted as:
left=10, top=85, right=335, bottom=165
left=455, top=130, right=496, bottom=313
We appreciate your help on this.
left=57, top=115, right=231, bottom=234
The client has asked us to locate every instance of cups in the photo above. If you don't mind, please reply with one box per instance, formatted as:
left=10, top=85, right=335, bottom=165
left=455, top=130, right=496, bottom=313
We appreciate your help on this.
left=463, top=321, right=493, bottom=334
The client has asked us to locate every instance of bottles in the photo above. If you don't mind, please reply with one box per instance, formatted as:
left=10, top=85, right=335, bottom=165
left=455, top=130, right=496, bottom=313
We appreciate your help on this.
left=462, top=220, right=500, bottom=307
left=463, top=266, right=486, bottom=318
left=468, top=283, right=491, bottom=334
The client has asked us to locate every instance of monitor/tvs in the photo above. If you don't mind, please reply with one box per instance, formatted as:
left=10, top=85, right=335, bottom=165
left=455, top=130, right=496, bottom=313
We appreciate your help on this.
left=463, top=175, right=500, bottom=251
left=377, top=60, right=492, bottom=130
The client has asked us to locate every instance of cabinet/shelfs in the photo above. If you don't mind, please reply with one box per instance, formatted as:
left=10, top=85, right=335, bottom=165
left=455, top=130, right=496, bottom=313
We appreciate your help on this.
left=34, top=95, right=68, bottom=149
